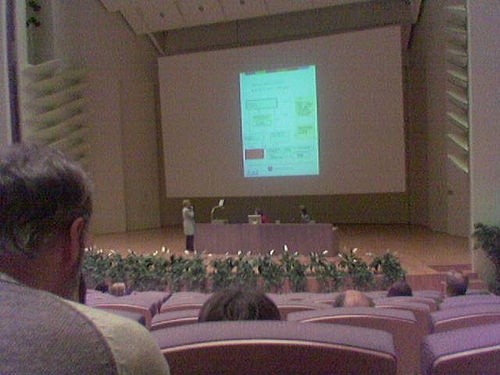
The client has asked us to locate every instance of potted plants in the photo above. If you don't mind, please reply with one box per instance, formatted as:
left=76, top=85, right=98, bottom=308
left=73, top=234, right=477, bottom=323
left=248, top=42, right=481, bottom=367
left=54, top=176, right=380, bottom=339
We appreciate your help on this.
left=470, top=222, right=500, bottom=296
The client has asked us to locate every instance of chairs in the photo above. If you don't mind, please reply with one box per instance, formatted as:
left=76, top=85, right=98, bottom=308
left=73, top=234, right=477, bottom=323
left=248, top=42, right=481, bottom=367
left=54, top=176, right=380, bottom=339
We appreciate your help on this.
left=85, top=290, right=500, bottom=375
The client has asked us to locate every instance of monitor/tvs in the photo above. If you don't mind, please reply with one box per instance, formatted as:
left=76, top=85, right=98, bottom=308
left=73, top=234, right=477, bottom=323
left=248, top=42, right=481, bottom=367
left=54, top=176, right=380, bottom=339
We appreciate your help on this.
left=248, top=215, right=262, bottom=224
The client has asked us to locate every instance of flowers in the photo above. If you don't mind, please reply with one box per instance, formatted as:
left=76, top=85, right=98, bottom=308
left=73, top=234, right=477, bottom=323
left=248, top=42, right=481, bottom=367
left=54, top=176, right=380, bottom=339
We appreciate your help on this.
left=80, top=245, right=406, bottom=292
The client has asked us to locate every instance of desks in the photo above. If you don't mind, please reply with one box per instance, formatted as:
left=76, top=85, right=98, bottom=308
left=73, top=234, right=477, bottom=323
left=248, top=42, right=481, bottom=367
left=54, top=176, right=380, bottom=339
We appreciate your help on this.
left=194, top=223, right=339, bottom=257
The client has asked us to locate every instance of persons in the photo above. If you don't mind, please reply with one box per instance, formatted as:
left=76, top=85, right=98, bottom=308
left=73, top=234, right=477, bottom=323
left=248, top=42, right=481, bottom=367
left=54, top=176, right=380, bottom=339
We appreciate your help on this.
left=0, top=143, right=170, bottom=374
left=111, top=282, right=126, bottom=296
left=255, top=209, right=266, bottom=223
left=446, top=271, right=468, bottom=295
left=301, top=208, right=310, bottom=223
left=387, top=281, right=411, bottom=297
left=333, top=289, right=376, bottom=308
left=182, top=199, right=195, bottom=254
left=197, top=287, right=281, bottom=323
left=96, top=283, right=108, bottom=293
